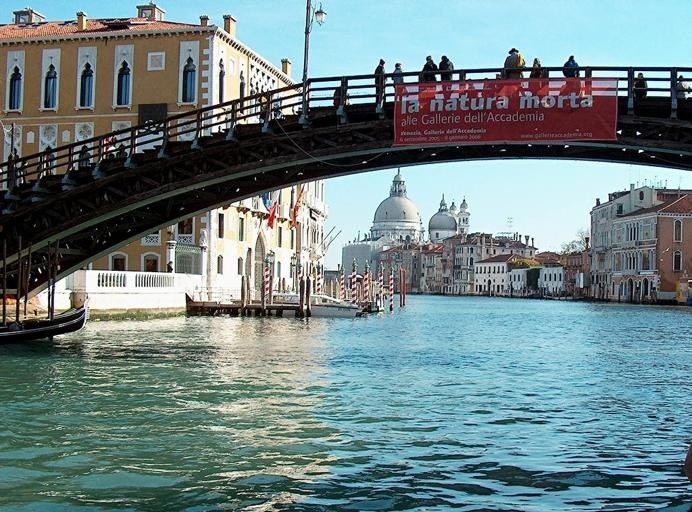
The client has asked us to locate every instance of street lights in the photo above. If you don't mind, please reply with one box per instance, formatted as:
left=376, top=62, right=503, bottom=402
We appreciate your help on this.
left=301, top=2, right=326, bottom=112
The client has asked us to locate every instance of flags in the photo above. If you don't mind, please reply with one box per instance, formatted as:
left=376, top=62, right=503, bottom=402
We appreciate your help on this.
left=290, top=185, right=304, bottom=228
left=267, top=201, right=277, bottom=229
left=261, top=192, right=272, bottom=211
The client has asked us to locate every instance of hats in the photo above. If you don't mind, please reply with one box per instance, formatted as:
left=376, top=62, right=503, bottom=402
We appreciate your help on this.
left=508, top=47, right=520, bottom=55
left=379, top=58, right=386, bottom=65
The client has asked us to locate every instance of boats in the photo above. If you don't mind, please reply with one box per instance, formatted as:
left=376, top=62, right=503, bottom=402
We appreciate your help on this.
left=0, top=290, right=86, bottom=347
left=270, top=293, right=365, bottom=318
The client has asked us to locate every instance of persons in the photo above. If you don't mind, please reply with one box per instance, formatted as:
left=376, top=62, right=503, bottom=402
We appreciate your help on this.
left=374, top=48, right=581, bottom=104
left=676, top=75, right=691, bottom=98
left=166, top=261, right=173, bottom=273
left=258, top=95, right=267, bottom=118
left=635, top=73, right=647, bottom=96
